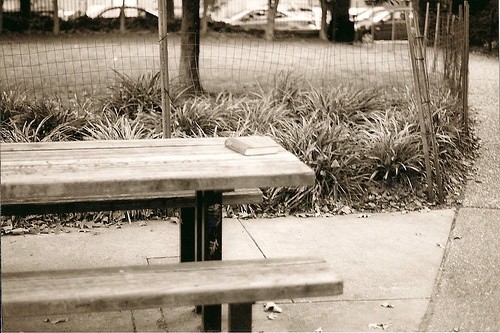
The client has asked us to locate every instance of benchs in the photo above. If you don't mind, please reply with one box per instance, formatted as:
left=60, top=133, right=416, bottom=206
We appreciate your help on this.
left=1, top=257, right=343, bottom=332
left=0, top=188, right=263, bottom=262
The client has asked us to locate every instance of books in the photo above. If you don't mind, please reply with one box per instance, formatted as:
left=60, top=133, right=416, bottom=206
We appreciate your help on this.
left=225, top=136, right=280, bottom=156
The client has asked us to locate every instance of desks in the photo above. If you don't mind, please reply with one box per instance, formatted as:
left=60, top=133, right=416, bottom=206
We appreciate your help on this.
left=1, top=135, right=316, bottom=332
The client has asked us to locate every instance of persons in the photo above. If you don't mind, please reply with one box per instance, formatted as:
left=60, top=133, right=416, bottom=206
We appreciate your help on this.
left=319, top=1, right=354, bottom=43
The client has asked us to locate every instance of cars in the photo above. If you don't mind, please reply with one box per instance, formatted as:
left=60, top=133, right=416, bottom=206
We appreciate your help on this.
left=93, top=7, right=159, bottom=29
left=224, top=8, right=321, bottom=32
left=355, top=9, right=413, bottom=44
